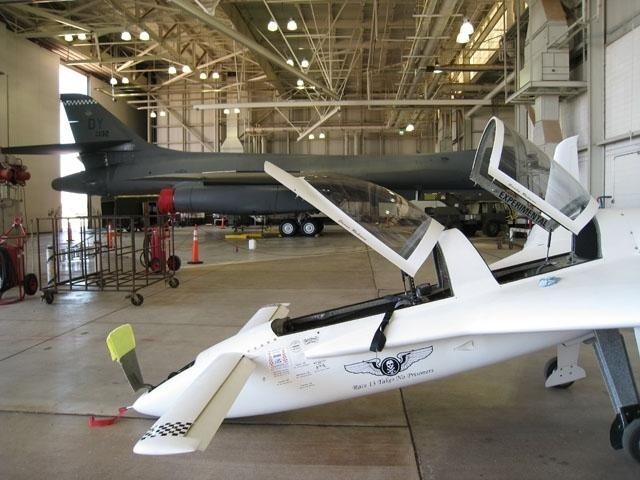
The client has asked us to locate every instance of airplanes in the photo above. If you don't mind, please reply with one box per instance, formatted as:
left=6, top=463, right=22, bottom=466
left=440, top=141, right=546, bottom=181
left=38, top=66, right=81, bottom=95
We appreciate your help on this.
left=105, top=116, right=639, bottom=461
left=0, top=92, right=544, bottom=240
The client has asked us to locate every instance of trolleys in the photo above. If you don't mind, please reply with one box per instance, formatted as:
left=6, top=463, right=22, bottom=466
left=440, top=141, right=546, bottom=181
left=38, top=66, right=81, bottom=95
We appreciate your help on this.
left=0, top=213, right=181, bottom=306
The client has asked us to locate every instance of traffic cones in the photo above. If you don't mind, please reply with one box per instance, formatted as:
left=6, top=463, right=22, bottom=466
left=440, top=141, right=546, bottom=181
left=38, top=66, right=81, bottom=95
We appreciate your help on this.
left=186, top=223, right=204, bottom=265
left=105, top=223, right=114, bottom=248
